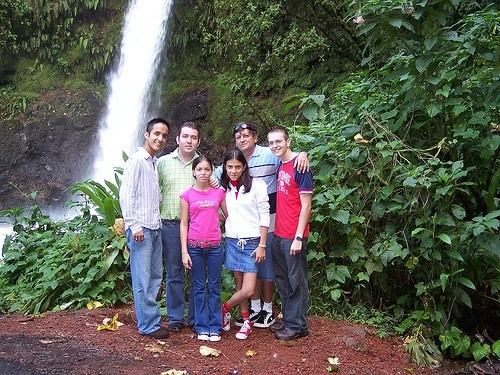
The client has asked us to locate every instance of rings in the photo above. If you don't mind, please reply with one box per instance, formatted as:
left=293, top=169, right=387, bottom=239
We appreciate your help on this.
left=136, top=239, right=137, bottom=241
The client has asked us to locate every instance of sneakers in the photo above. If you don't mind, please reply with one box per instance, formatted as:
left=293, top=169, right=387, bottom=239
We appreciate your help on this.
left=210, top=330, right=221, bottom=341
left=198, top=331, right=209, bottom=341
left=235, top=309, right=262, bottom=327
left=236, top=319, right=253, bottom=340
left=254, top=309, right=275, bottom=328
left=221, top=304, right=232, bottom=331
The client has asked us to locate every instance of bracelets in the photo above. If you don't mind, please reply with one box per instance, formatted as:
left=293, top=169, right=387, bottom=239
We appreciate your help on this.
left=259, top=245, right=266, bottom=248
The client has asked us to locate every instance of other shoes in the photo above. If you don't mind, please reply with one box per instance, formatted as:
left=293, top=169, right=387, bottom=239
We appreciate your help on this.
left=192, top=323, right=196, bottom=331
left=275, top=327, right=308, bottom=340
left=146, top=329, right=169, bottom=339
left=270, top=319, right=286, bottom=332
left=170, top=323, right=182, bottom=332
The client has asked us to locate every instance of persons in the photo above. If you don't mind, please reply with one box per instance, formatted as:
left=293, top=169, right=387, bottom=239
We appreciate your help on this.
left=119, top=118, right=172, bottom=339
left=219, top=147, right=271, bottom=340
left=214, top=121, right=310, bottom=327
left=179, top=155, right=229, bottom=341
left=267, top=127, right=314, bottom=340
left=157, top=121, right=221, bottom=333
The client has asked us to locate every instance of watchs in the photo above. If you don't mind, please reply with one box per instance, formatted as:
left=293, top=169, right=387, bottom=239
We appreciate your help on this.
left=295, top=236, right=303, bottom=241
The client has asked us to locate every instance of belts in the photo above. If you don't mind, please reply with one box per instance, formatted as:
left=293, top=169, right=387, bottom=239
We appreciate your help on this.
left=187, top=239, right=221, bottom=248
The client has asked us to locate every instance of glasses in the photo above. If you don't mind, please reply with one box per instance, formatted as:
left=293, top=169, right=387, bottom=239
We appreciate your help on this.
left=233, top=123, right=256, bottom=133
